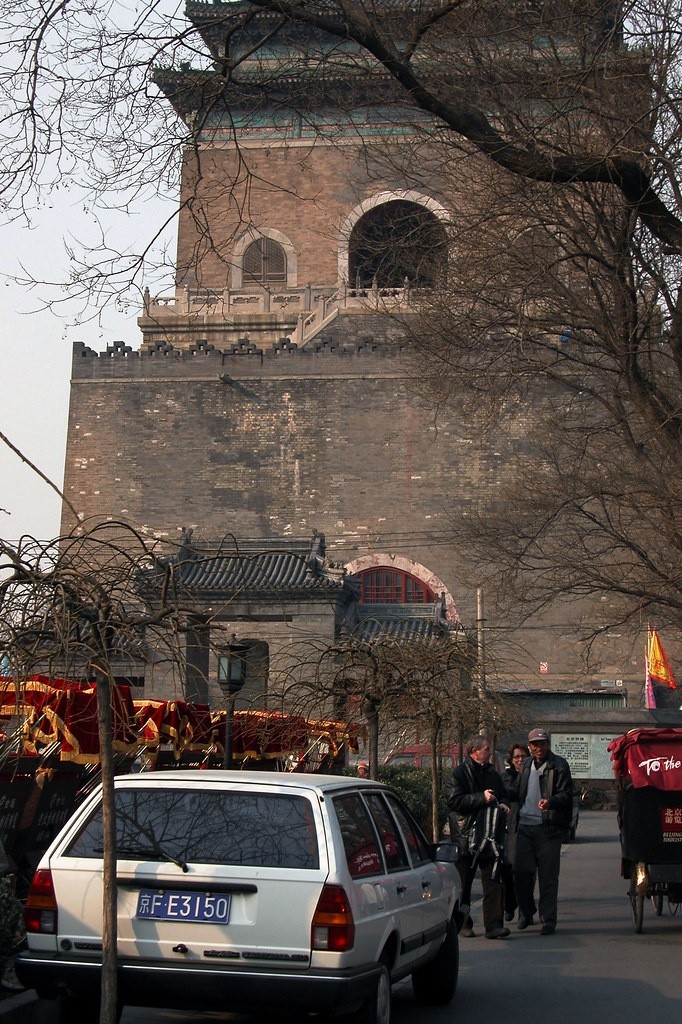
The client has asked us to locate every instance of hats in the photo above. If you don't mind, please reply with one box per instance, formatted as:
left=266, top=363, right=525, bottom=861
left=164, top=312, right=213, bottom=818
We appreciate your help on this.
left=528, top=728, right=549, bottom=742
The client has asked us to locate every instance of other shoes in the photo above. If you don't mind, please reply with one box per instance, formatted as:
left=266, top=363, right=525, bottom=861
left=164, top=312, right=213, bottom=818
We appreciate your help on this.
left=517, top=915, right=534, bottom=929
left=504, top=904, right=516, bottom=921
left=459, top=927, right=475, bottom=938
left=539, top=924, right=557, bottom=936
left=484, top=927, right=511, bottom=939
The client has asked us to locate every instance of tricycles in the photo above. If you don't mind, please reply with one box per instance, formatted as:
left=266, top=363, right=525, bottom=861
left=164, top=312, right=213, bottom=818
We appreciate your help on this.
left=0, top=674, right=363, bottom=786
left=606, top=726, right=682, bottom=936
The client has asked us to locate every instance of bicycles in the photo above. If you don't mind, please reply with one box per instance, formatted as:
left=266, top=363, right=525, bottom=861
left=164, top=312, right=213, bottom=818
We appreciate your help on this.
left=578, top=781, right=607, bottom=811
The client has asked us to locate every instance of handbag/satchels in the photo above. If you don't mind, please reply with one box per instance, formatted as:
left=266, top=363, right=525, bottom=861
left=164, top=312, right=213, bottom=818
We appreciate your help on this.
left=441, top=814, right=471, bottom=838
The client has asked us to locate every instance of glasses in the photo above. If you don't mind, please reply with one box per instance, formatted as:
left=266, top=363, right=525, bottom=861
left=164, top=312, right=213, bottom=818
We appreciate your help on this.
left=512, top=754, right=530, bottom=759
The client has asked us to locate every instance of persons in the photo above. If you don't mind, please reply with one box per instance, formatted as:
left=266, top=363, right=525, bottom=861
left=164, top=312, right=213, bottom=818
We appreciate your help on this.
left=358, top=764, right=369, bottom=777
left=447, top=729, right=574, bottom=938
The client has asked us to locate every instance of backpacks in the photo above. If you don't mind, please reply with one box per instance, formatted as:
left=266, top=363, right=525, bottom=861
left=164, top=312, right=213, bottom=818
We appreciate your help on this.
left=468, top=795, right=507, bottom=861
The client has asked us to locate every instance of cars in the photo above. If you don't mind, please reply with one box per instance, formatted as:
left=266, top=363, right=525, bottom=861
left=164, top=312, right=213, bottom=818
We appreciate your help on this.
left=563, top=781, right=583, bottom=843
left=12, top=770, right=470, bottom=1024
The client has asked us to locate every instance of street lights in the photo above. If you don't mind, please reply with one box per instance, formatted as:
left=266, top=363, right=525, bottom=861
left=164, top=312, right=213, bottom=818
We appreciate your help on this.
left=214, top=633, right=251, bottom=770
